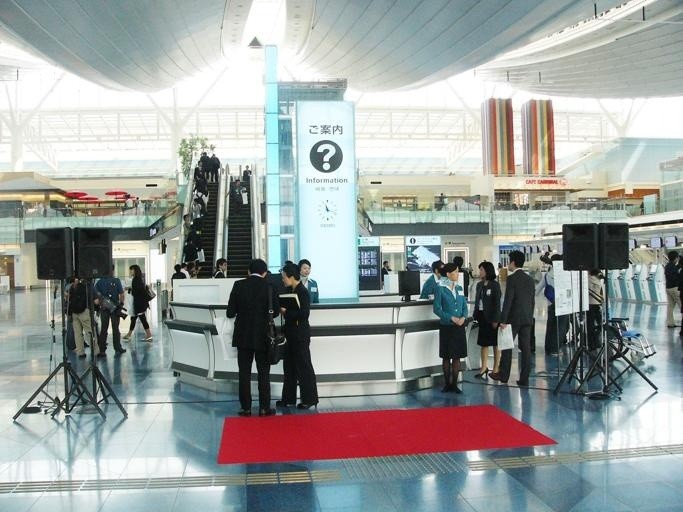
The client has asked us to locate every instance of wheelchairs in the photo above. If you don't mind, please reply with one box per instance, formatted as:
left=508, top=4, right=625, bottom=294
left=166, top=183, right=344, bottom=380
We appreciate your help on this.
left=591, top=312, right=657, bottom=363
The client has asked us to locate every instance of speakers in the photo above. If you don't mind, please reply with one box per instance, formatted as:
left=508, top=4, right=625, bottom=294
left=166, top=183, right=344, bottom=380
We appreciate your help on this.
left=598, top=223, right=629, bottom=270
left=74, top=227, right=112, bottom=279
left=562, top=223, right=597, bottom=271
left=36, top=227, right=74, bottom=280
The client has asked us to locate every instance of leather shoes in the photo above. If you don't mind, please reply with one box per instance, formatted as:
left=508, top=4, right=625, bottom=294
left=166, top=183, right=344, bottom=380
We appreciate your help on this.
left=238, top=408, right=275, bottom=416
left=489, top=373, right=529, bottom=386
left=441, top=384, right=462, bottom=394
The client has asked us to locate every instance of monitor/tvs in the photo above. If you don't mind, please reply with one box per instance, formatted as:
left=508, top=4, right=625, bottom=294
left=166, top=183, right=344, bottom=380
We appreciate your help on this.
left=664, top=236, right=677, bottom=247
left=247, top=274, right=285, bottom=290
left=629, top=239, right=637, bottom=248
left=650, top=237, right=662, bottom=247
left=398, top=271, right=420, bottom=301
left=519, top=246, right=539, bottom=253
left=542, top=245, right=550, bottom=252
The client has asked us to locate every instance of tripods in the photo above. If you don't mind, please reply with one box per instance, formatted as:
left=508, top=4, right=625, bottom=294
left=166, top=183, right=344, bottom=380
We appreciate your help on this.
left=51, top=279, right=129, bottom=419
left=13, top=279, right=107, bottom=421
left=576, top=270, right=659, bottom=395
left=554, top=270, right=623, bottom=396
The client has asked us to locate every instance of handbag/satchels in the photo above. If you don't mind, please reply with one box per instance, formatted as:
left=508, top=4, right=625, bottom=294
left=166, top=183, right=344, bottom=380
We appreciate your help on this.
left=144, top=284, right=155, bottom=301
left=543, top=284, right=554, bottom=304
left=262, top=327, right=288, bottom=364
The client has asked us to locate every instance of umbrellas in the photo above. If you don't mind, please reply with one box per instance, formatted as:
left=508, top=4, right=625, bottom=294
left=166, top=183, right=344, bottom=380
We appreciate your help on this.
left=64, top=191, right=139, bottom=204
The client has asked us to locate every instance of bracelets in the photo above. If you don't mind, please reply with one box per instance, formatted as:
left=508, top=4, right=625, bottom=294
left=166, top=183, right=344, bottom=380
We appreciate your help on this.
left=462, top=316, right=466, bottom=321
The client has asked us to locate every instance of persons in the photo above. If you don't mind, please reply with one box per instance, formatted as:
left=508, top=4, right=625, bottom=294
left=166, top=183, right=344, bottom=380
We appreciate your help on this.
left=664, top=251, right=682, bottom=328
left=69, top=277, right=100, bottom=358
left=94, top=264, right=126, bottom=357
left=122, top=265, right=153, bottom=342
left=453, top=256, right=470, bottom=297
left=277, top=259, right=319, bottom=410
left=382, top=261, right=392, bottom=282
left=226, top=258, right=280, bottom=417
left=488, top=250, right=535, bottom=386
left=121, top=194, right=133, bottom=213
left=473, top=261, right=502, bottom=378
left=434, top=263, right=469, bottom=394
left=439, top=193, right=448, bottom=203
left=419, top=260, right=445, bottom=299
left=64, top=278, right=89, bottom=350
left=588, top=269, right=605, bottom=349
left=171, top=152, right=251, bottom=302
left=134, top=197, right=139, bottom=208
left=534, top=254, right=570, bottom=357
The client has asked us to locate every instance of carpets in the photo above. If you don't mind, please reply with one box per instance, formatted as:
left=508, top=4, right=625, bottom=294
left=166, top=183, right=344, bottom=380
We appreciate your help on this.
left=217, top=403, right=559, bottom=464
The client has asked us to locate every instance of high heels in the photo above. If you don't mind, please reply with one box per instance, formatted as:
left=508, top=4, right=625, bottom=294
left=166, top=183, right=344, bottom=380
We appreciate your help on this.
left=474, top=367, right=489, bottom=377
left=276, top=399, right=319, bottom=409
left=122, top=334, right=153, bottom=342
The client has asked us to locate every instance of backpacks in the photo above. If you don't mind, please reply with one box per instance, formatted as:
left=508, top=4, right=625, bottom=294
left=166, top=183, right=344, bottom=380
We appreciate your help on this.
left=71, top=282, right=87, bottom=313
